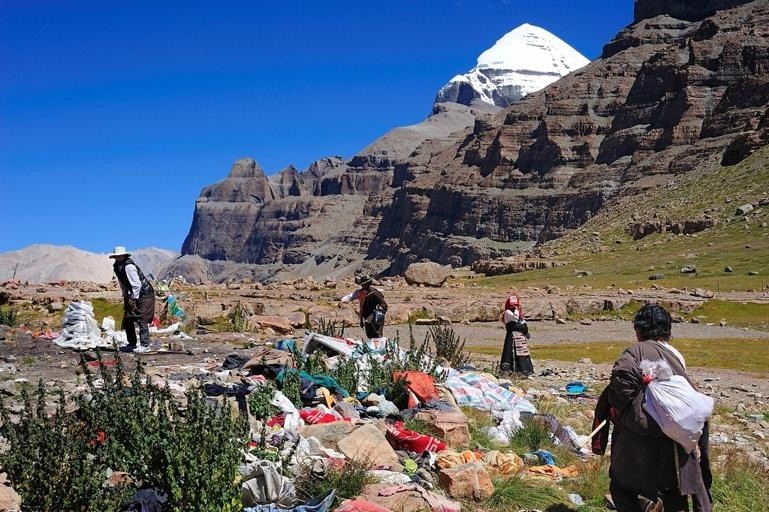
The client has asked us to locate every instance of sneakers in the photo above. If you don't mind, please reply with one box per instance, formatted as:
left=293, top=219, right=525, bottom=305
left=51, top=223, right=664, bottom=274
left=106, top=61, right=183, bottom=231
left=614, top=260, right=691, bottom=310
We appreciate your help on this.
left=132, top=345, right=152, bottom=353
left=120, top=344, right=137, bottom=352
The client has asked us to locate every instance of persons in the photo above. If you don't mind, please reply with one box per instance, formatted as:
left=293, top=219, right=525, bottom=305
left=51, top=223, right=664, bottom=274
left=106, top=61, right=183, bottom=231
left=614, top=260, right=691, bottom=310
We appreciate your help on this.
left=110, top=247, right=155, bottom=353
left=501, top=294, right=533, bottom=373
left=356, top=277, right=388, bottom=338
left=607, top=302, right=713, bottom=511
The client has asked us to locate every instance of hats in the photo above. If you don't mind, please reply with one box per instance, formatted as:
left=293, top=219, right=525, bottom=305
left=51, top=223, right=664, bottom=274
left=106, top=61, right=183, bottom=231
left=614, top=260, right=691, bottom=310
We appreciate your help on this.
left=109, top=246, right=132, bottom=259
left=357, top=273, right=372, bottom=286
left=508, top=295, right=518, bottom=305
